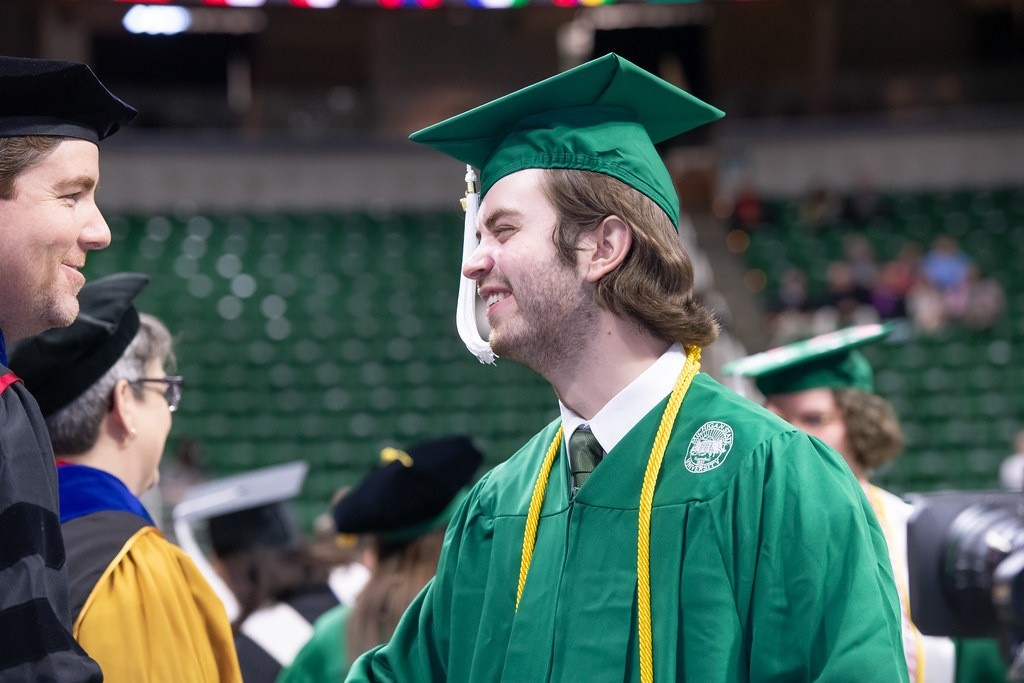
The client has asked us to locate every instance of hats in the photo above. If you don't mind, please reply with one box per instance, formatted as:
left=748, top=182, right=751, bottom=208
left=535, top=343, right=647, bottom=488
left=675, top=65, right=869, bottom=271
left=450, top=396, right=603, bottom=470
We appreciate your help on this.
left=330, top=429, right=486, bottom=563
left=170, top=455, right=310, bottom=560
left=0, top=55, right=139, bottom=151
left=721, top=322, right=897, bottom=394
left=5, top=268, right=149, bottom=419
left=406, top=51, right=726, bottom=235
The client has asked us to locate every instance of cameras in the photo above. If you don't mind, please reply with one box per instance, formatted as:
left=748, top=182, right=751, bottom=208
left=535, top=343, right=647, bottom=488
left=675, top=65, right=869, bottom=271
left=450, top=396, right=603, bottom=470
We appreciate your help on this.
left=906, top=493, right=1024, bottom=639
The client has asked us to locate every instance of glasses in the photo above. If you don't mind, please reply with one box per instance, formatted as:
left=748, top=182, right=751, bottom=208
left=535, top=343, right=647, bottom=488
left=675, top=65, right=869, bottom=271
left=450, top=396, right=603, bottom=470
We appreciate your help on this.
left=107, top=374, right=184, bottom=413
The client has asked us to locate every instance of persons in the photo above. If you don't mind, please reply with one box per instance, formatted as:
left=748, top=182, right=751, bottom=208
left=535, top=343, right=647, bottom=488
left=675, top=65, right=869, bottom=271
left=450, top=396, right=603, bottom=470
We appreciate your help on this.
left=34, top=308, right=243, bottom=683
left=757, top=346, right=958, bottom=682
left=273, top=456, right=472, bottom=683
left=1, top=96, right=113, bottom=683
left=201, top=486, right=372, bottom=683
left=706, top=149, right=1007, bottom=354
left=322, top=108, right=909, bottom=682
